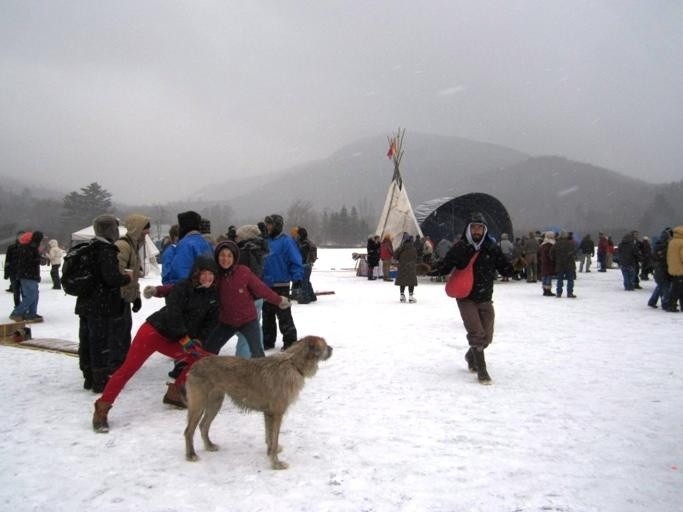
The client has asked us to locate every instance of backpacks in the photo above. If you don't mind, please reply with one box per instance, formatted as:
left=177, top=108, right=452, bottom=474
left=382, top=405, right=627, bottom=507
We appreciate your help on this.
left=60, top=240, right=100, bottom=296
left=302, top=240, right=317, bottom=263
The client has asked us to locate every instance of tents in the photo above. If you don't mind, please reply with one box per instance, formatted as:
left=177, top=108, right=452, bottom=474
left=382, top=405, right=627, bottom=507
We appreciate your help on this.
left=374, top=180, right=423, bottom=276
left=412, top=193, right=515, bottom=249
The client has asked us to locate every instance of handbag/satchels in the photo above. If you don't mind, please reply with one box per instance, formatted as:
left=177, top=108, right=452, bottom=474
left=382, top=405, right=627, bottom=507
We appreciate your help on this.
left=446, top=269, right=473, bottom=298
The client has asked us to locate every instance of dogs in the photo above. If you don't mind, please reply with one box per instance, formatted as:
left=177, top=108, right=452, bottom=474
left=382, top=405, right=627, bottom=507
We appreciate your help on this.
left=183, top=336, right=333, bottom=470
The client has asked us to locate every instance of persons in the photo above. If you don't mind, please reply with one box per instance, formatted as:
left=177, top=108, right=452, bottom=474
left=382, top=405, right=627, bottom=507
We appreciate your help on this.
left=488, top=228, right=595, bottom=298
left=419, top=211, right=528, bottom=385
left=4, top=231, right=51, bottom=323
left=110, top=215, right=151, bottom=373
left=75, top=215, right=134, bottom=392
left=46, top=239, right=63, bottom=289
left=143, top=211, right=318, bottom=368
left=93, top=256, right=220, bottom=434
left=368, top=231, right=463, bottom=303
left=597, top=225, right=682, bottom=312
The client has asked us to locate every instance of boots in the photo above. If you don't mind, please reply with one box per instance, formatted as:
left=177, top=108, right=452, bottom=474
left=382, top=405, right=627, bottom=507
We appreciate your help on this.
left=93, top=398, right=112, bottom=431
left=465, top=345, right=492, bottom=384
left=625, top=282, right=642, bottom=290
left=164, top=383, right=187, bottom=407
left=5, top=285, right=12, bottom=292
left=542, top=286, right=576, bottom=298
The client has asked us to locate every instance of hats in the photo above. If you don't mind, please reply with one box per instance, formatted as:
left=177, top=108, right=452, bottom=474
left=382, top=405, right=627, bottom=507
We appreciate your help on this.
left=264, top=214, right=283, bottom=238
left=235, top=225, right=260, bottom=242
left=92, top=215, right=119, bottom=245
left=545, top=231, right=554, bottom=240
left=123, top=215, right=150, bottom=260
left=661, top=228, right=673, bottom=240
left=559, top=229, right=569, bottom=238
left=178, top=212, right=201, bottom=232
left=403, top=232, right=409, bottom=240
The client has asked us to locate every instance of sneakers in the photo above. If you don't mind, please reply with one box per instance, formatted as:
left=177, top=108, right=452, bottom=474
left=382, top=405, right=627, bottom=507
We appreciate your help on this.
left=368, top=274, right=394, bottom=281
left=83, top=378, right=108, bottom=391
left=502, top=276, right=537, bottom=283
left=400, top=293, right=416, bottom=301
left=579, top=269, right=606, bottom=272
left=648, top=299, right=683, bottom=312
left=300, top=295, right=317, bottom=303
left=9, top=312, right=43, bottom=323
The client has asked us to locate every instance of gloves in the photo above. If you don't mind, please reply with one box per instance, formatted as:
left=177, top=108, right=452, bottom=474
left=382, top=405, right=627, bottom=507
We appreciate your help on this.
left=145, top=286, right=156, bottom=298
left=292, top=280, right=302, bottom=289
left=181, top=336, right=201, bottom=355
left=279, top=296, right=292, bottom=308
left=131, top=298, right=141, bottom=312
